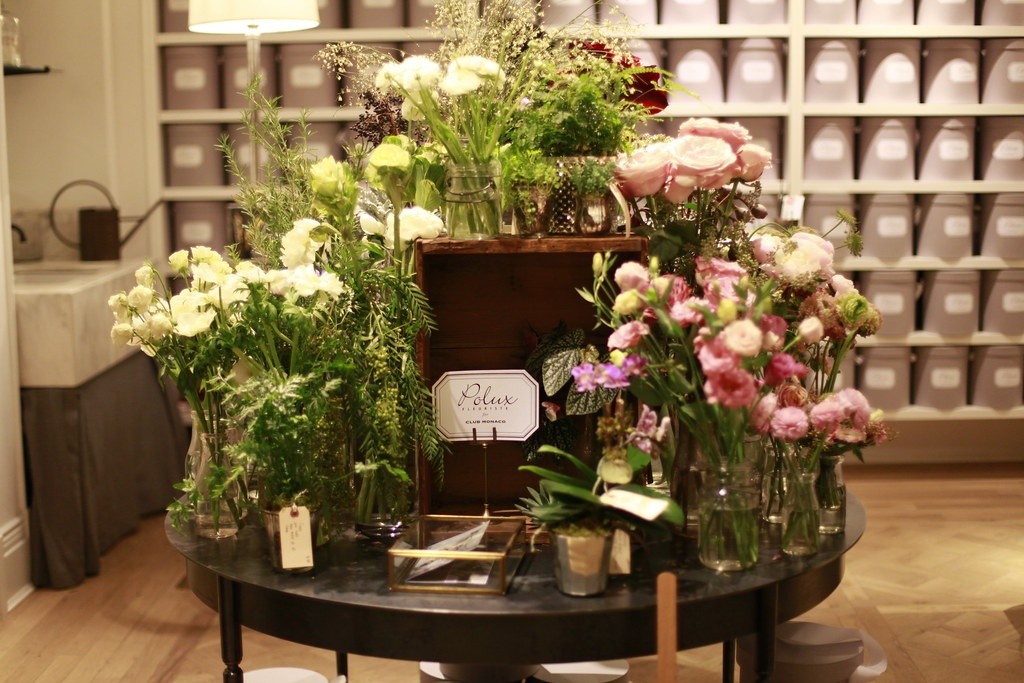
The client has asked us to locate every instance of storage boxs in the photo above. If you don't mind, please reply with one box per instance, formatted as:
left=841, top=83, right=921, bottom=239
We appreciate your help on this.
left=412, top=235, right=647, bottom=550
left=390, top=516, right=528, bottom=595
left=158, top=0, right=1024, bottom=412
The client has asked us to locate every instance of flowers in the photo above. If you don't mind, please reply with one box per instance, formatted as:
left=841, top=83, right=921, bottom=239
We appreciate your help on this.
left=516, top=445, right=685, bottom=549
left=107, top=1, right=887, bottom=534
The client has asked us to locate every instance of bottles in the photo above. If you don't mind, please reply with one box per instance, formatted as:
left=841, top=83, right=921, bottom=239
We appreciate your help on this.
left=0, top=0, right=25, bottom=67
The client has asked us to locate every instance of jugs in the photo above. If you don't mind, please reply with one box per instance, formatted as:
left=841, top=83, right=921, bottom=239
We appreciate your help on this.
left=47, top=180, right=167, bottom=261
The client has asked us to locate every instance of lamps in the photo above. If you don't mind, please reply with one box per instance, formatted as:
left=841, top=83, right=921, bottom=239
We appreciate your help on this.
left=188, top=0, right=319, bottom=179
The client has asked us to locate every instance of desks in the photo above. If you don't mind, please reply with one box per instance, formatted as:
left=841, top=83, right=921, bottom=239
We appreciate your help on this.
left=165, top=488, right=866, bottom=683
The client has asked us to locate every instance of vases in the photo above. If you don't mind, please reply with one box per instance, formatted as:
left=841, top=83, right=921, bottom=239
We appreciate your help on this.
left=551, top=523, right=613, bottom=595
left=185, top=409, right=847, bottom=572
left=514, top=156, right=618, bottom=237
left=444, top=161, right=501, bottom=241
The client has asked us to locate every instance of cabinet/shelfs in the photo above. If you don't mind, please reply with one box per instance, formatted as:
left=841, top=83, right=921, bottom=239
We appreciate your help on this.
left=141, top=0, right=1024, bottom=466
